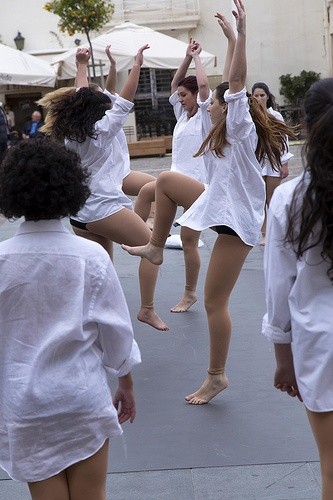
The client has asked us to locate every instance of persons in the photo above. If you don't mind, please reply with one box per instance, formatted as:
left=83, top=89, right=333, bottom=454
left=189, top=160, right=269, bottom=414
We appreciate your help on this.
left=243, top=81, right=296, bottom=245
left=132, top=33, right=209, bottom=315
left=41, top=42, right=171, bottom=331
left=0, top=139, right=142, bottom=500
left=0, top=101, right=45, bottom=163
left=261, top=77, right=333, bottom=499
left=64, top=42, right=115, bottom=266
left=121, top=0, right=267, bottom=407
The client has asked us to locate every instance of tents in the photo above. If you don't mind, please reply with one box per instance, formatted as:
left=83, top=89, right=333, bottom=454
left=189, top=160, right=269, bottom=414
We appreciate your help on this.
left=53, top=18, right=217, bottom=158
left=0, top=42, right=56, bottom=89
